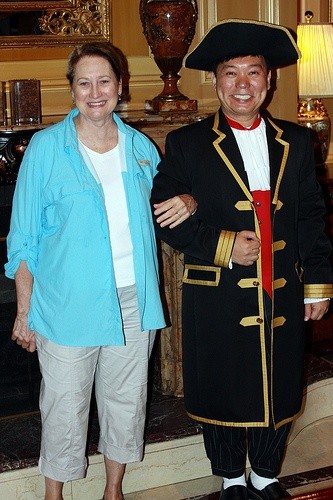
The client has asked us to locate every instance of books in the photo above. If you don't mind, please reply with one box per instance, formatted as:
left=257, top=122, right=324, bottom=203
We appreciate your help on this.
left=0, top=78, right=41, bottom=127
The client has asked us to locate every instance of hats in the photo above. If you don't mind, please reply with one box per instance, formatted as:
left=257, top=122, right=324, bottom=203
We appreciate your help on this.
left=182, top=18, right=302, bottom=72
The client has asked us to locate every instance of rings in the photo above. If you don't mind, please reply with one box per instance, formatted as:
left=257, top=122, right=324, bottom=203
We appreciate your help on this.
left=176, top=212, right=182, bottom=218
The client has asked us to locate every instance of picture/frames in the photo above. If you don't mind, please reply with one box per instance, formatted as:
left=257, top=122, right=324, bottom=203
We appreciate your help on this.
left=0, top=0, right=112, bottom=48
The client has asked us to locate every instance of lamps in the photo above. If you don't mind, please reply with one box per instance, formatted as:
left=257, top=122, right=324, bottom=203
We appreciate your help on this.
left=296, top=23, right=333, bottom=162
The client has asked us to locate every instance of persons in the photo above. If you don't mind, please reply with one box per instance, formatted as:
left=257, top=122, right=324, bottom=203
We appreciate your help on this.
left=4, top=42, right=199, bottom=500
left=150, top=17, right=333, bottom=500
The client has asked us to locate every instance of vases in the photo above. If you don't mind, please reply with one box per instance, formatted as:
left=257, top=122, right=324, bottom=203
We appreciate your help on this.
left=138, top=0, right=198, bottom=101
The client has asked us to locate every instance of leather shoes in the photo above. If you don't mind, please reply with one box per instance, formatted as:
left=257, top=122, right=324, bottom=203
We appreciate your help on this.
left=247, top=472, right=292, bottom=500
left=219, top=480, right=249, bottom=500
